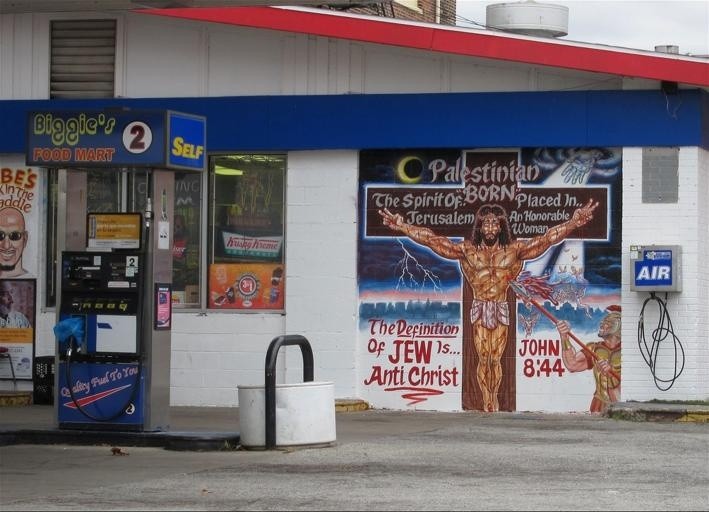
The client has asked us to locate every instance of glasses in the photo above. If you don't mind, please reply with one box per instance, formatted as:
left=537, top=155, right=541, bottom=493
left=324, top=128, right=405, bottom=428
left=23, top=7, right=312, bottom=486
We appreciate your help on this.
left=0, top=230, right=28, bottom=241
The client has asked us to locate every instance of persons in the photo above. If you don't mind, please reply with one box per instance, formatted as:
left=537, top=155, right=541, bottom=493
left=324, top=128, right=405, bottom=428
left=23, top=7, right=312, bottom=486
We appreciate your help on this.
left=0, top=280, right=31, bottom=328
left=0, top=207, right=32, bottom=278
left=556, top=305, right=621, bottom=413
left=378, top=199, right=600, bottom=413
